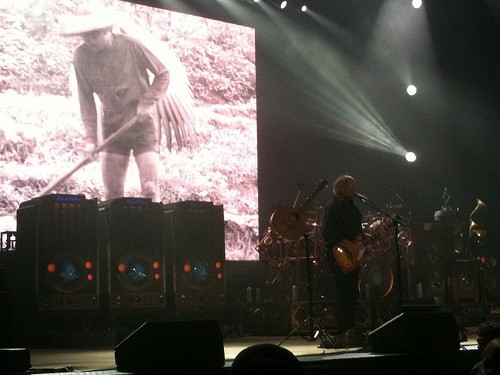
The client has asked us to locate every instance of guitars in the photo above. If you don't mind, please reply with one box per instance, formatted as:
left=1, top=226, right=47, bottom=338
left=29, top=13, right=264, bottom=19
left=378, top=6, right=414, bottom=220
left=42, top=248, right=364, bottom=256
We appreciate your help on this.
left=270, top=179, right=330, bottom=242
left=334, top=215, right=401, bottom=276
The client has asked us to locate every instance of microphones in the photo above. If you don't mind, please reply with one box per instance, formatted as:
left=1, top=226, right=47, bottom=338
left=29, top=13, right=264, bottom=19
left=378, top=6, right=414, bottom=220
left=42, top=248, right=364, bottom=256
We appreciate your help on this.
left=353, top=192, right=367, bottom=200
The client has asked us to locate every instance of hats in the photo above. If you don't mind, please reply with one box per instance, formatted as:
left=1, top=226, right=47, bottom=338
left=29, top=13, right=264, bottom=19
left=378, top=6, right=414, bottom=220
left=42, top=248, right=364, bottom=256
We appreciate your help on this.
left=57, top=0, right=125, bottom=36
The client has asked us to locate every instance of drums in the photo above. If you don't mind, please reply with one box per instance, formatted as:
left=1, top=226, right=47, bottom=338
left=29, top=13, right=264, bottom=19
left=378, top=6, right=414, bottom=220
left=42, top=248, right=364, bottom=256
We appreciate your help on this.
left=358, top=260, right=395, bottom=298
left=285, top=254, right=316, bottom=280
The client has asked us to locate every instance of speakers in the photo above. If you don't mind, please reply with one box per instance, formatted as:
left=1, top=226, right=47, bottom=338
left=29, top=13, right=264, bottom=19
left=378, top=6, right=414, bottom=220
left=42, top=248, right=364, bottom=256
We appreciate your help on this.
left=114, top=319, right=225, bottom=372
left=368, top=305, right=460, bottom=351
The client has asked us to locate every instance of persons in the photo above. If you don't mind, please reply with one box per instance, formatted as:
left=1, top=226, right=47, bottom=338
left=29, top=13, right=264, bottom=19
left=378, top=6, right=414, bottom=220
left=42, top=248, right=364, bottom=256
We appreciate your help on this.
left=59, top=4, right=169, bottom=202
left=319, top=174, right=363, bottom=332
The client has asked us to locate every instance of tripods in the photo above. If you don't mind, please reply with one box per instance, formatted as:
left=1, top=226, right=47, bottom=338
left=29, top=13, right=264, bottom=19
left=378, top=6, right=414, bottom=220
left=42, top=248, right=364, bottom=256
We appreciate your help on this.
left=276, top=234, right=335, bottom=349
